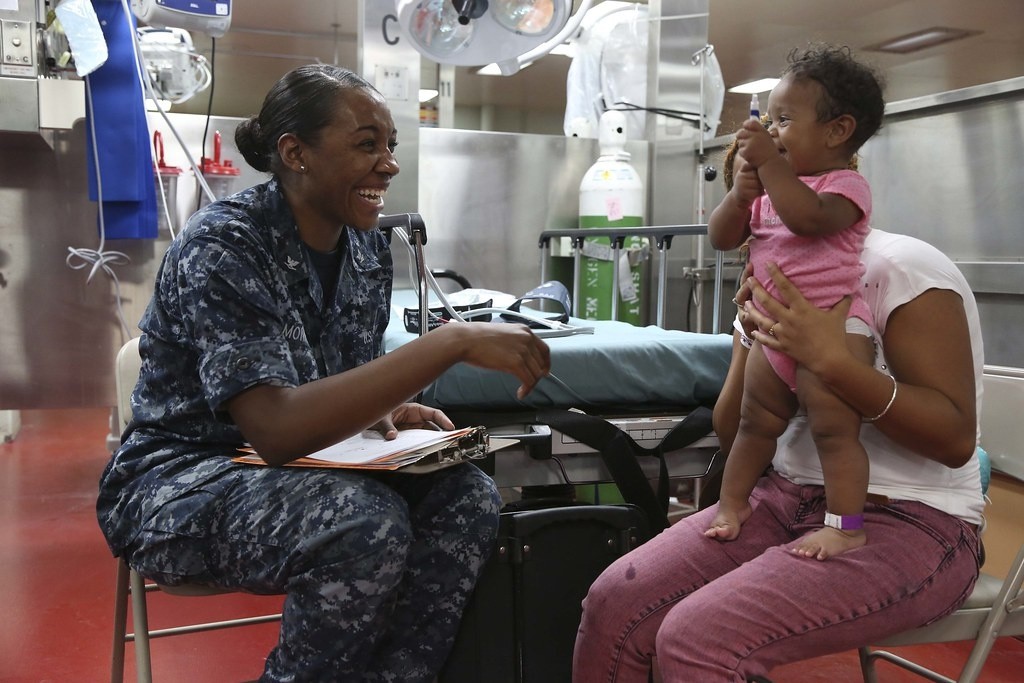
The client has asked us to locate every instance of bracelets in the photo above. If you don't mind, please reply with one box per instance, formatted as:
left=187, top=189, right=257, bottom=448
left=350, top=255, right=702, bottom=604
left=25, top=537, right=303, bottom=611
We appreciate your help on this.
left=739, top=334, right=753, bottom=349
left=859, top=372, right=896, bottom=424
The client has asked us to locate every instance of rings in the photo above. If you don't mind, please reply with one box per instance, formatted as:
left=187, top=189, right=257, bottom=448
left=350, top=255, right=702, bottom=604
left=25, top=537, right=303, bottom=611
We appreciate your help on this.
left=732, top=297, right=744, bottom=309
left=768, top=321, right=778, bottom=336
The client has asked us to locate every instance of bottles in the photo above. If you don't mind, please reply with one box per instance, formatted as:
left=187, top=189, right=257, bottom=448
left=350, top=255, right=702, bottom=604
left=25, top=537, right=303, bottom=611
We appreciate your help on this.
left=578, top=111, right=646, bottom=327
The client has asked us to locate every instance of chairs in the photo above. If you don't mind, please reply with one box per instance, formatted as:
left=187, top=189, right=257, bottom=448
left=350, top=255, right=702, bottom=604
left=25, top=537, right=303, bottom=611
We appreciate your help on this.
left=858, top=365, right=1024, bottom=683
left=110, top=334, right=283, bottom=683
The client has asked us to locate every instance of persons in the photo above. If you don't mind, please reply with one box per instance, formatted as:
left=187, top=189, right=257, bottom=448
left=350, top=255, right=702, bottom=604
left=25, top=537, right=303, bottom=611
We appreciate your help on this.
left=570, top=114, right=986, bottom=682
left=700, top=45, right=886, bottom=559
left=94, top=62, right=553, bottom=682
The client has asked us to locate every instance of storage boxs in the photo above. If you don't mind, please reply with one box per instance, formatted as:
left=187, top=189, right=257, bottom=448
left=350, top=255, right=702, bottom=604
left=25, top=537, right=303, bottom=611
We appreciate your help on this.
left=576, top=217, right=650, bottom=327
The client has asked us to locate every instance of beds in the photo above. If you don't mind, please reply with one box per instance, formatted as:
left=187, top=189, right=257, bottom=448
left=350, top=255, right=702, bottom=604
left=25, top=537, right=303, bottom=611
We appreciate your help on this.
left=380, top=221, right=736, bottom=542
